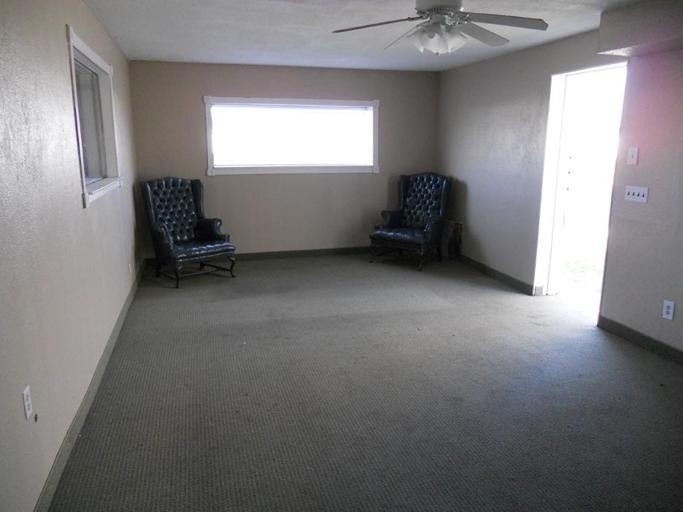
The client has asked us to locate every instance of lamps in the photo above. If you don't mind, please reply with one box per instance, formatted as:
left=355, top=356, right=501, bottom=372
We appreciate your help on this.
left=407, top=21, right=456, bottom=55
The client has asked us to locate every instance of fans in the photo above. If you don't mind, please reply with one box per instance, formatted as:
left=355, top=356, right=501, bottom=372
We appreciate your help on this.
left=333, top=0, right=549, bottom=49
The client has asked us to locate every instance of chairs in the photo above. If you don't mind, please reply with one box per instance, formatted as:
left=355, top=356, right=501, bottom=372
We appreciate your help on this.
left=142, top=176, right=237, bottom=288
left=369, top=172, right=461, bottom=271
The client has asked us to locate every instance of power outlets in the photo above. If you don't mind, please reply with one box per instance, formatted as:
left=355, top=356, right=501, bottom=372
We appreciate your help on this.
left=662, top=300, right=674, bottom=319
